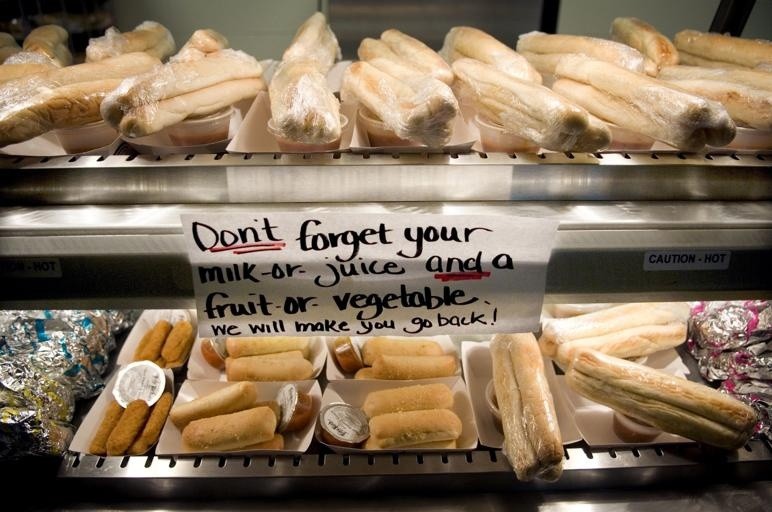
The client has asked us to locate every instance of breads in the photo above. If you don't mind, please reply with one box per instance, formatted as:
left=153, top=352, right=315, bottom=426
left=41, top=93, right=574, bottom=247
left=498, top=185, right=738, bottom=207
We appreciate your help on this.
left=90, top=320, right=193, bottom=457
left=0, top=11, right=772, bottom=155
left=490, top=331, right=563, bottom=485
left=360, top=338, right=464, bottom=451
left=536, top=300, right=758, bottom=453
left=169, top=335, right=315, bottom=452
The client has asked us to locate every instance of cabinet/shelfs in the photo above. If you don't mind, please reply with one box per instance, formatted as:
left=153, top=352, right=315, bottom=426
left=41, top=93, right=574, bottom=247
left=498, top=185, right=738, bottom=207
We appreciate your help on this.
left=0, top=133, right=771, bottom=509
